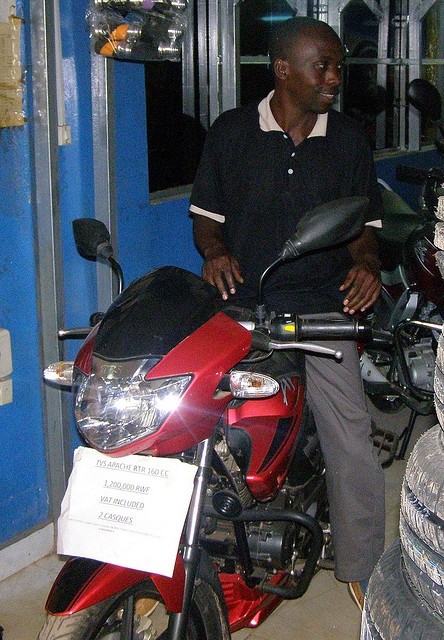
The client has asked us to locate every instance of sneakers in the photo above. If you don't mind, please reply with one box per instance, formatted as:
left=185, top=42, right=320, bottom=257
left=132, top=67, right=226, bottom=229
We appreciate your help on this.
left=348, top=566, right=401, bottom=640
left=132, top=597, right=158, bottom=615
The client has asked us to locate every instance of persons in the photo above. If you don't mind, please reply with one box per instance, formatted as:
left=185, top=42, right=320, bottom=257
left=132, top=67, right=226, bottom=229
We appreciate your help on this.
left=188, top=17, right=385, bottom=574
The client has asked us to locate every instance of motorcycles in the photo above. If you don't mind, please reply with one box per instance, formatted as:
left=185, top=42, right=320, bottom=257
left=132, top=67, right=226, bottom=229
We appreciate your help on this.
left=36, top=195, right=398, bottom=640
left=357, top=79, right=443, bottom=461
